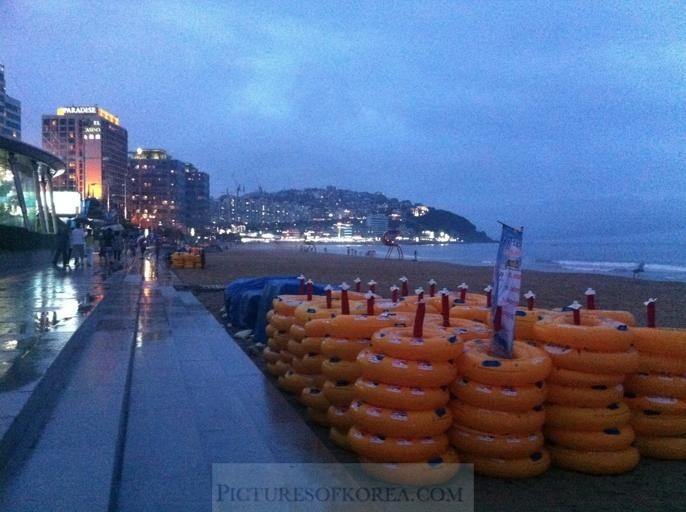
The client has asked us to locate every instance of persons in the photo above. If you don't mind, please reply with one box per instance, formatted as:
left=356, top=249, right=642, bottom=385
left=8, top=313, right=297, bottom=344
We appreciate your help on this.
left=155, top=239, right=160, bottom=261
left=51, top=220, right=72, bottom=269
left=71, top=222, right=86, bottom=265
left=127, top=235, right=135, bottom=259
left=99, top=239, right=108, bottom=266
left=84, top=228, right=95, bottom=268
left=105, top=228, right=114, bottom=260
left=121, top=235, right=127, bottom=257
left=113, top=230, right=121, bottom=261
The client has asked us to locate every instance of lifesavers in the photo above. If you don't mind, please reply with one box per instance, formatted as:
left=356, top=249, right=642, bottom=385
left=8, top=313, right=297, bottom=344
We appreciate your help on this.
left=301, top=388, right=325, bottom=408
left=286, top=340, right=304, bottom=357
left=327, top=406, right=352, bottom=431
left=633, top=436, right=686, bottom=460
left=546, top=403, right=631, bottom=431
left=329, top=426, right=348, bottom=449
left=543, top=425, right=634, bottom=453
left=449, top=427, right=544, bottom=459
left=263, top=346, right=278, bottom=362
left=274, top=330, right=289, bottom=348
left=284, top=371, right=310, bottom=394
left=457, top=449, right=550, bottom=479
left=447, top=399, right=547, bottom=436
left=294, top=299, right=359, bottom=327
left=632, top=413, right=686, bottom=439
left=279, top=349, right=293, bottom=363
left=321, top=337, right=370, bottom=361
left=457, top=338, right=552, bottom=387
left=322, top=380, right=354, bottom=406
left=546, top=385, right=624, bottom=408
left=356, top=347, right=458, bottom=388
left=625, top=373, right=686, bottom=399
left=330, top=286, right=556, bottom=331
left=305, top=319, right=330, bottom=337
left=633, top=327, right=686, bottom=355
left=265, top=324, right=277, bottom=338
left=301, top=336, right=322, bottom=354
left=289, top=323, right=307, bottom=342
left=548, top=444, right=640, bottom=474
left=172, top=247, right=201, bottom=268
left=276, top=299, right=299, bottom=316
left=372, top=325, right=465, bottom=362
left=638, top=350, right=686, bottom=377
left=277, top=377, right=297, bottom=393
left=347, top=427, right=448, bottom=463
left=268, top=335, right=282, bottom=353
left=326, top=315, right=411, bottom=340
left=349, top=400, right=452, bottom=436
left=579, top=309, right=636, bottom=327
left=266, top=362, right=279, bottom=377
left=354, top=378, right=449, bottom=411
left=266, top=310, right=274, bottom=323
left=273, top=294, right=326, bottom=311
left=292, top=356, right=304, bottom=373
left=452, top=376, right=549, bottom=411
left=322, top=358, right=360, bottom=384
left=532, top=311, right=633, bottom=352
left=271, top=314, right=292, bottom=331
left=301, top=356, right=323, bottom=376
left=542, top=344, right=640, bottom=375
left=548, top=367, right=625, bottom=389
left=313, top=411, right=327, bottom=424
left=360, top=449, right=461, bottom=485
left=628, top=395, right=685, bottom=416
left=311, top=372, right=326, bottom=387
